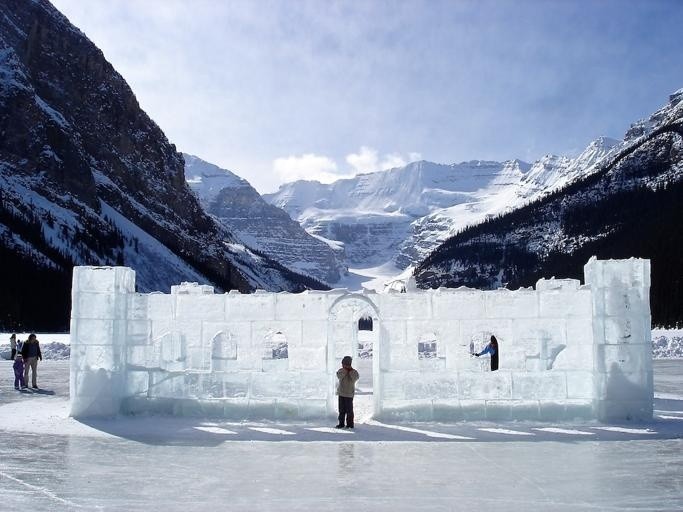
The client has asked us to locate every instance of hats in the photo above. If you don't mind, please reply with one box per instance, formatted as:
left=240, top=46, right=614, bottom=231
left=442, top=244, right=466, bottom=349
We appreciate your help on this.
left=342, top=356, right=352, bottom=365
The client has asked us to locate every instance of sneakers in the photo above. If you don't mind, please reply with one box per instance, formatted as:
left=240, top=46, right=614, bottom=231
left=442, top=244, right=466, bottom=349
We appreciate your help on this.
left=336, top=424, right=354, bottom=429
left=14, top=386, right=37, bottom=390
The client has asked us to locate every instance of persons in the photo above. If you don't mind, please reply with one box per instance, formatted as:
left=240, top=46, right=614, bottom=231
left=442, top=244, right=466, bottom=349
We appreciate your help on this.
left=9, top=333, right=17, bottom=360
left=12, top=355, right=27, bottom=390
left=470, top=335, right=498, bottom=373
left=16, top=340, right=23, bottom=355
left=20, top=333, right=42, bottom=390
left=334, top=355, right=359, bottom=430
